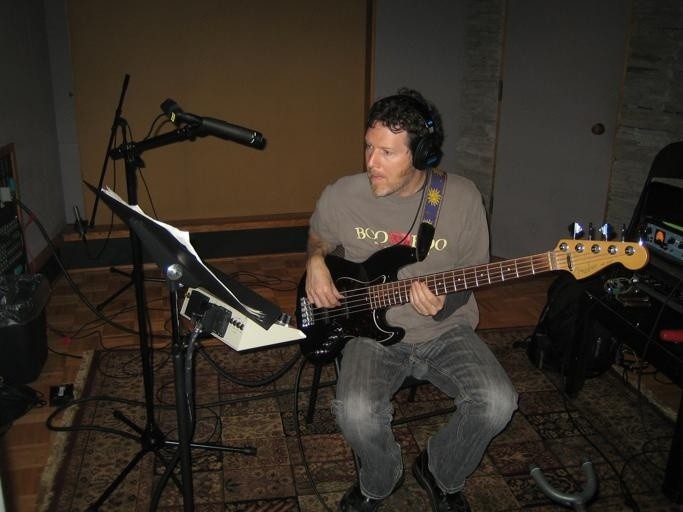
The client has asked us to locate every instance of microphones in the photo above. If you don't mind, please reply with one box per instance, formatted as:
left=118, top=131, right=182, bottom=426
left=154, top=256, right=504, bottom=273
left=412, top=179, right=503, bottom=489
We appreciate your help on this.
left=172, top=110, right=264, bottom=148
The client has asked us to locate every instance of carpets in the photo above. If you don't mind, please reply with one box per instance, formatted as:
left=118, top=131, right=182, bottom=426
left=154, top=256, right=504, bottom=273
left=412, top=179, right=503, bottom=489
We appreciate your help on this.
left=34, top=325, right=682, bottom=512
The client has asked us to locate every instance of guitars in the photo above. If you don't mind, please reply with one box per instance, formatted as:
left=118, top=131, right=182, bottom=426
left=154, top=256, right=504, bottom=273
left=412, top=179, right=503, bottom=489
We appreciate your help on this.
left=297, top=223, right=650, bottom=366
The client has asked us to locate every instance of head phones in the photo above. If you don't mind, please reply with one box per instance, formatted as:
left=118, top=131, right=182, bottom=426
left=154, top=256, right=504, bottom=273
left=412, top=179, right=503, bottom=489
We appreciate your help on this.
left=387, top=94, right=444, bottom=171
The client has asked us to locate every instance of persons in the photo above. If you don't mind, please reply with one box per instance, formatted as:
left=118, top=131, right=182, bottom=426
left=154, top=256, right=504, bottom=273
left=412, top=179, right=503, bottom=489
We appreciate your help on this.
left=301, top=85, right=520, bottom=512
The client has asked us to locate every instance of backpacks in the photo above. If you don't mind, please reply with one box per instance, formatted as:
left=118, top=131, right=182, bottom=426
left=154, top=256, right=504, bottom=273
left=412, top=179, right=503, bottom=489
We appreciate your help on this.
left=524, top=267, right=620, bottom=383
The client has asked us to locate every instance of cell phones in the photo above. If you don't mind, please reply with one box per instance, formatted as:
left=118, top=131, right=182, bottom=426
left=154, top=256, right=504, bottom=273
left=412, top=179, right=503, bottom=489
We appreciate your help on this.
left=618, top=291, right=649, bottom=302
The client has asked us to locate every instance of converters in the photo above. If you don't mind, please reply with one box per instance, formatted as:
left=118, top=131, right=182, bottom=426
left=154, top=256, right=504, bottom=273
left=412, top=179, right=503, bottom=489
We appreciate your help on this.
left=48, top=384, right=75, bottom=406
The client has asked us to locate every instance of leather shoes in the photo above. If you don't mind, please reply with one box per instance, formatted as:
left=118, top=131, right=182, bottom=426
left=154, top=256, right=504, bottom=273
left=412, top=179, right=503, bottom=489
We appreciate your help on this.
left=411, top=447, right=472, bottom=512
left=337, top=466, right=406, bottom=512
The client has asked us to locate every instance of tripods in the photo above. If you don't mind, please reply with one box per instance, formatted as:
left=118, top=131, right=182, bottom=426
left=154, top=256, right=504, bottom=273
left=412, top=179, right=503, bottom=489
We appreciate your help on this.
left=84, top=143, right=259, bottom=512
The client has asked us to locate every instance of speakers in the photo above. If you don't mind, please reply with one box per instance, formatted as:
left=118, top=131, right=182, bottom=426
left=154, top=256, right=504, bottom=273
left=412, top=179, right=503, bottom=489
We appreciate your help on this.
left=0, top=142, right=32, bottom=275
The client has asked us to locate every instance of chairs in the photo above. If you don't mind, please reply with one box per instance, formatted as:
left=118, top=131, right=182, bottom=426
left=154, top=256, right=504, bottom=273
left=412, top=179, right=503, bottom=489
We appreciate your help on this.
left=305, top=352, right=457, bottom=485
